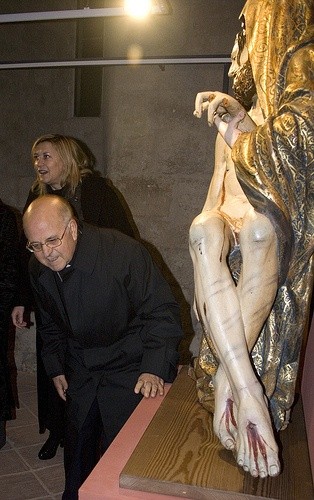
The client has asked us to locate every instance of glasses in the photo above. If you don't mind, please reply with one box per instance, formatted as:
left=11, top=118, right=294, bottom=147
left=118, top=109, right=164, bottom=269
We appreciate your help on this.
left=26, top=217, right=73, bottom=253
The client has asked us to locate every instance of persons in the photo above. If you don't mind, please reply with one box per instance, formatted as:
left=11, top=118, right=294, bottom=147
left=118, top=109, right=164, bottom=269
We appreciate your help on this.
left=22, top=193, right=180, bottom=500
left=0, top=198, right=21, bottom=450
left=11, top=133, right=136, bottom=460
left=187, top=0, right=314, bottom=477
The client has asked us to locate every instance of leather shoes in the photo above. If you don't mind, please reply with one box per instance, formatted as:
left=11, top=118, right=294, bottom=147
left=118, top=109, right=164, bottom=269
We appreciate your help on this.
left=38, top=429, right=61, bottom=460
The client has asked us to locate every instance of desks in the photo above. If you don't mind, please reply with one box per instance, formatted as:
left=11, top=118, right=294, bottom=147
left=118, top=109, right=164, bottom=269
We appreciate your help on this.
left=78, top=365, right=314, bottom=500
